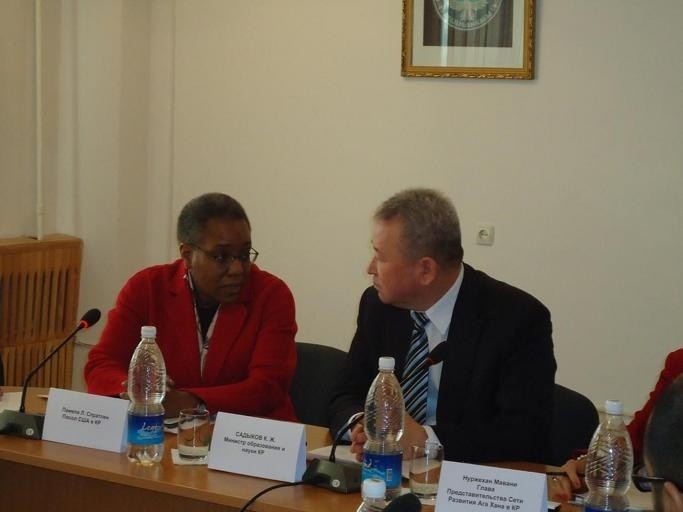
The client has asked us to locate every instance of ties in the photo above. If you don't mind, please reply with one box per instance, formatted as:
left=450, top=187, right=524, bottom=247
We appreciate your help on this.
left=400, top=312, right=430, bottom=425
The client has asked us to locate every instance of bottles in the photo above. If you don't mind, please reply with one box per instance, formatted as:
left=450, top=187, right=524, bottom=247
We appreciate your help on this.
left=124, top=323, right=167, bottom=466
left=358, top=354, right=406, bottom=505
left=356, top=479, right=389, bottom=512
left=580, top=398, right=634, bottom=511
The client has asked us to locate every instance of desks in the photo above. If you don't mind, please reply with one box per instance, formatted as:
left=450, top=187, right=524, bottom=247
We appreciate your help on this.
left=0, top=383, right=589, bottom=512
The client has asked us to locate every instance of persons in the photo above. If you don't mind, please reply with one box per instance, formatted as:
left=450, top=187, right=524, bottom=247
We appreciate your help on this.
left=631, top=372, right=682, bottom=512
left=552, top=347, right=682, bottom=499
left=326, top=186, right=557, bottom=458
left=85, top=193, right=299, bottom=424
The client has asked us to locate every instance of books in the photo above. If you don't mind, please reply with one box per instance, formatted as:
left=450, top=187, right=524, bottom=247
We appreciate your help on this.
left=306, top=441, right=442, bottom=479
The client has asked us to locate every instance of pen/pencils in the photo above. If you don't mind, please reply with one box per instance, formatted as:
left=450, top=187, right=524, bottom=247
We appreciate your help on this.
left=546, top=472, right=586, bottom=476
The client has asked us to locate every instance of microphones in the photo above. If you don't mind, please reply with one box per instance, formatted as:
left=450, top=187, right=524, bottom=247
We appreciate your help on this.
left=302, top=341, right=454, bottom=493
left=0, top=309, right=101, bottom=440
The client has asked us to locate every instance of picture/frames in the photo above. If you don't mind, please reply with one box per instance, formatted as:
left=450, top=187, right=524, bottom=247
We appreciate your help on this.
left=399, top=0, right=537, bottom=80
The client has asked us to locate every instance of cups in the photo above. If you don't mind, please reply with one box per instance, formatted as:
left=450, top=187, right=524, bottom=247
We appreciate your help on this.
left=176, top=408, right=211, bottom=459
left=410, top=438, right=445, bottom=499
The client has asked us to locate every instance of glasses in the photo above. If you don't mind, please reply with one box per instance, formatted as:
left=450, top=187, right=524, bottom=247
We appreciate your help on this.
left=632, top=463, right=665, bottom=492
left=195, top=245, right=259, bottom=265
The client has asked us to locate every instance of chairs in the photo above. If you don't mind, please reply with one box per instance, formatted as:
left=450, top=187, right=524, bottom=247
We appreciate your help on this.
left=290, top=341, right=352, bottom=430
left=542, top=383, right=600, bottom=468
